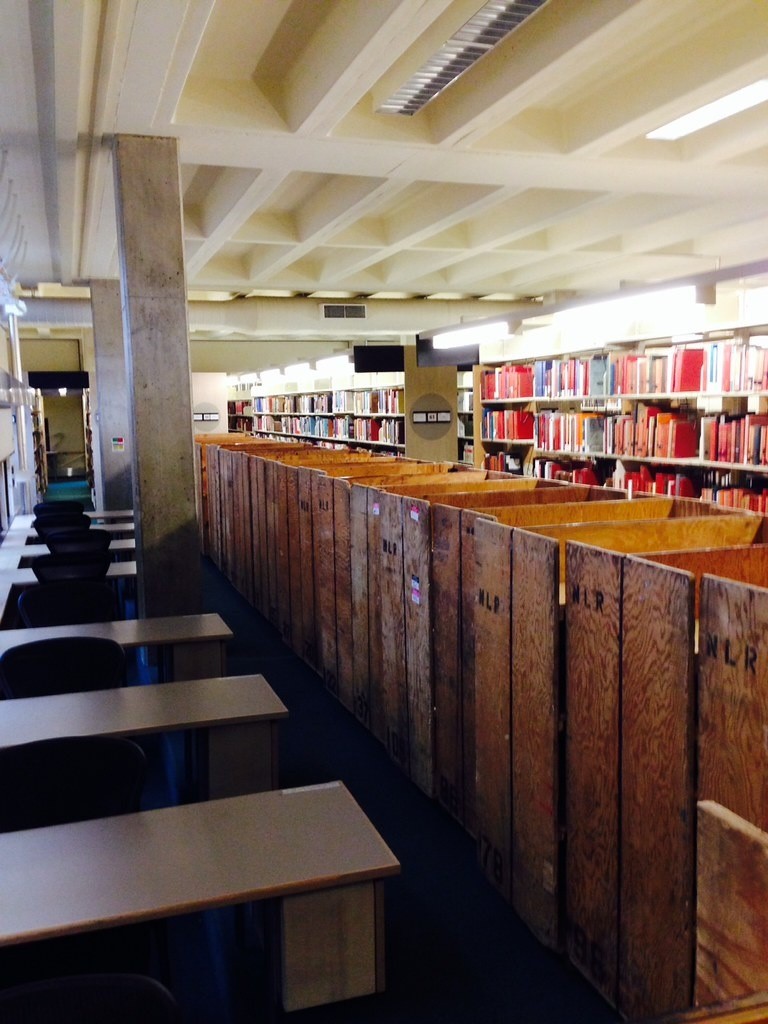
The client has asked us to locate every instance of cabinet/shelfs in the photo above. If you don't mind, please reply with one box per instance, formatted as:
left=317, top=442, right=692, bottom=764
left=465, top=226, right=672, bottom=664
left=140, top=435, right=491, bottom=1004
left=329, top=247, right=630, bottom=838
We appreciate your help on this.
left=225, top=321, right=768, bottom=516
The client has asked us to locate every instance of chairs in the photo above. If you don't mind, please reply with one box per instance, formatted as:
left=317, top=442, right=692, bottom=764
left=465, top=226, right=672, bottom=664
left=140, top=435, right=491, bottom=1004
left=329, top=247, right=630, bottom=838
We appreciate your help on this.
left=45, top=528, right=112, bottom=555
left=33, top=512, right=91, bottom=536
left=33, top=500, right=84, bottom=517
left=0, top=635, right=128, bottom=700
left=1, top=733, right=151, bottom=833
left=32, top=550, right=114, bottom=584
left=18, top=579, right=119, bottom=628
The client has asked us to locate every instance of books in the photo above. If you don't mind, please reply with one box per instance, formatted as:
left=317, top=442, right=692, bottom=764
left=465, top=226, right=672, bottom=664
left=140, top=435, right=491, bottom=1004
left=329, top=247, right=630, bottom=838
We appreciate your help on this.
left=229, top=387, right=406, bottom=455
left=457, top=389, right=475, bottom=464
left=483, top=337, right=768, bottom=518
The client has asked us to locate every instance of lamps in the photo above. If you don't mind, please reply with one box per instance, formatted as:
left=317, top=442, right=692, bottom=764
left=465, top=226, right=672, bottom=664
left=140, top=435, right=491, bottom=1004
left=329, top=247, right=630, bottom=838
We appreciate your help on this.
left=58, top=387, right=68, bottom=396
left=645, top=76, right=768, bottom=140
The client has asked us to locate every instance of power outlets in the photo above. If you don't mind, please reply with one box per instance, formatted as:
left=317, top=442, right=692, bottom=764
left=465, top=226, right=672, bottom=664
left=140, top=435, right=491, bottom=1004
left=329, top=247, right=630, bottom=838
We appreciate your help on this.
left=194, top=413, right=219, bottom=422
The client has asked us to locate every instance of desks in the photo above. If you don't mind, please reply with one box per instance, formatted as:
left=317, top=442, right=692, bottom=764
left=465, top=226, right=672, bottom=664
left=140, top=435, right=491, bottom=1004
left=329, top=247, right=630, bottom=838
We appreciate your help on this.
left=27, top=522, right=135, bottom=537
left=0, top=612, right=235, bottom=682
left=19, top=537, right=136, bottom=558
left=83, top=509, right=133, bottom=519
left=0, top=776, right=403, bottom=1012
left=11, top=559, right=137, bottom=586
left=0, top=673, right=290, bottom=801
